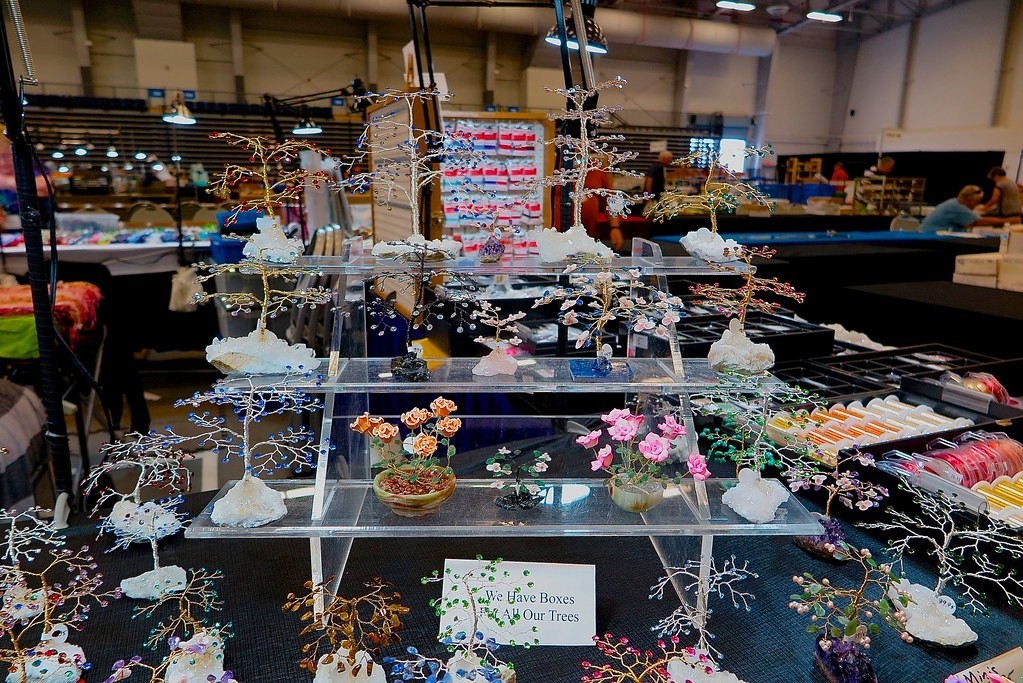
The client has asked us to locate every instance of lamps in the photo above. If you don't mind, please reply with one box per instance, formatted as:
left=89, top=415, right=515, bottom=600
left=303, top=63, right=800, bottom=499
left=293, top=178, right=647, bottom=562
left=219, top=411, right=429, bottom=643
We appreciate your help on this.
left=160, top=92, right=199, bottom=264
left=292, top=117, right=322, bottom=136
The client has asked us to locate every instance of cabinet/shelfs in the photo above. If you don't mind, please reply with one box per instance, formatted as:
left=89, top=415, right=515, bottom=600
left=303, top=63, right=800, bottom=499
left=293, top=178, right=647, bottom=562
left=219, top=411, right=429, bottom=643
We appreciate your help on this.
left=183, top=255, right=826, bottom=631
left=851, top=175, right=926, bottom=216
left=784, top=157, right=822, bottom=184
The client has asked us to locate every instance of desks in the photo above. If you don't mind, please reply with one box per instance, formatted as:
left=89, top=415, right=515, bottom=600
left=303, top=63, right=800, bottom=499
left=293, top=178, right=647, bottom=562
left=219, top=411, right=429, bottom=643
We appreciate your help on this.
left=650, top=211, right=1023, bottom=331
left=4, top=230, right=214, bottom=270
left=0, top=281, right=100, bottom=470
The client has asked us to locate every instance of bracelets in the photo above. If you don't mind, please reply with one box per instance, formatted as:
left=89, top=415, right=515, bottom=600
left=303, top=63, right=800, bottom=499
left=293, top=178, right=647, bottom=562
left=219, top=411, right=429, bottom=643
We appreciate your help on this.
left=609, top=226, right=620, bottom=229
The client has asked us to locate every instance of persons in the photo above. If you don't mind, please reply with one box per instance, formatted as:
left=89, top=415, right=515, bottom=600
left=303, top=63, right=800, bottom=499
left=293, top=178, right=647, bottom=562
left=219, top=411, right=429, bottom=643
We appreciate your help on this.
left=979, top=166, right=1023, bottom=217
left=89, top=292, right=157, bottom=439
left=829, top=161, right=849, bottom=205
left=570, top=152, right=623, bottom=253
left=857, top=157, right=895, bottom=212
left=645, top=151, right=673, bottom=221
left=919, top=185, right=1022, bottom=233
